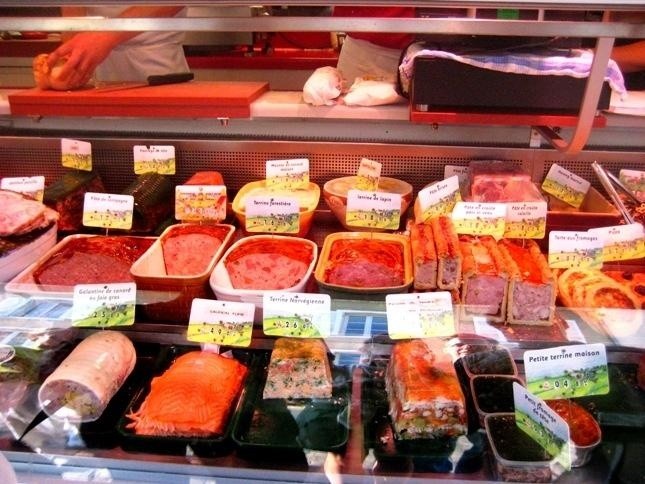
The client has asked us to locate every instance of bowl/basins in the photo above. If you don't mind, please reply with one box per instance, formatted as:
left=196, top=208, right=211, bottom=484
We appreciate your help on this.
left=323, top=175, right=412, bottom=232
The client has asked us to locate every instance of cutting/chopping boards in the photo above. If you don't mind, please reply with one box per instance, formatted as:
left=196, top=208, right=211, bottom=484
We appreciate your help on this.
left=6, top=80, right=270, bottom=118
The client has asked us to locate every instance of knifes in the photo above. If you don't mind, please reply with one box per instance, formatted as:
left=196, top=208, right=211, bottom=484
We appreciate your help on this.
left=96, top=71, right=194, bottom=92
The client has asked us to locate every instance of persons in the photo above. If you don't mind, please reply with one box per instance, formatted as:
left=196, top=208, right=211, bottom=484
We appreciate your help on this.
left=330, top=3, right=471, bottom=91
left=29, top=1, right=195, bottom=92
left=588, top=8, right=645, bottom=89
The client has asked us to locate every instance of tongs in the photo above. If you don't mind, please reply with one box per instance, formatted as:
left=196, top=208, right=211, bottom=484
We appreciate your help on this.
left=591, top=159, right=643, bottom=225
